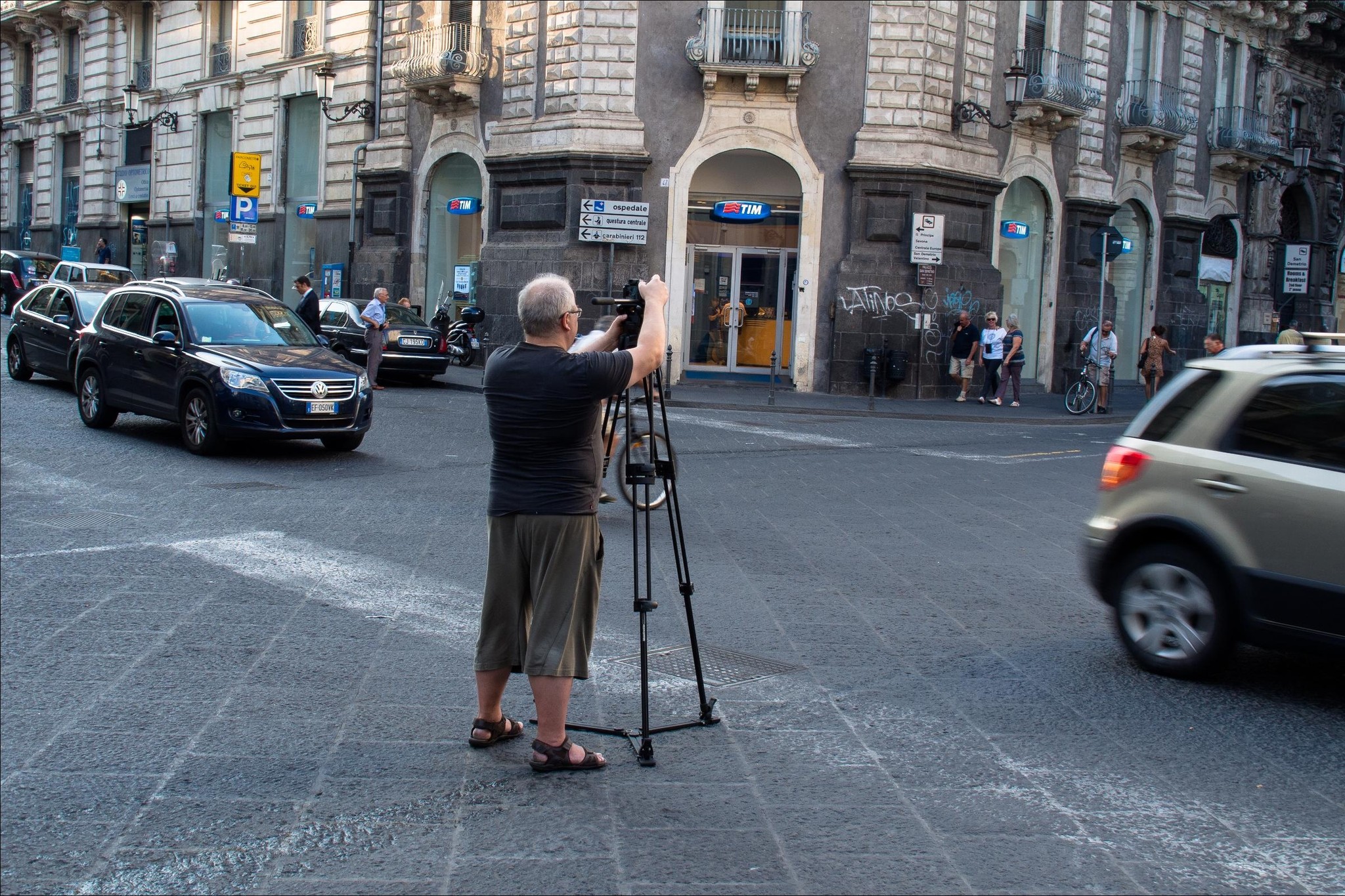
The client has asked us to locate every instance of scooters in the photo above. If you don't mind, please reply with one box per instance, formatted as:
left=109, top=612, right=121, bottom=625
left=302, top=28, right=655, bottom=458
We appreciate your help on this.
left=429, top=290, right=485, bottom=367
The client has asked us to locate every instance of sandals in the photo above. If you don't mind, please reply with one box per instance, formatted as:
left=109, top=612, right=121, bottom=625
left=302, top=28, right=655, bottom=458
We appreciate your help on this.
left=529, top=736, right=607, bottom=770
left=468, top=713, right=523, bottom=746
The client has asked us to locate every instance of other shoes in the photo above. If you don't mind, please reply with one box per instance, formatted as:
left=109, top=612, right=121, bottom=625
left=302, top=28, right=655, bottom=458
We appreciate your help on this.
left=977, top=397, right=985, bottom=404
left=1089, top=405, right=1100, bottom=414
left=706, top=360, right=717, bottom=364
left=987, top=399, right=1002, bottom=406
left=372, top=385, right=384, bottom=390
left=718, top=359, right=726, bottom=365
left=954, top=394, right=966, bottom=402
left=1098, top=406, right=1107, bottom=414
left=736, top=362, right=740, bottom=366
left=1009, top=402, right=1020, bottom=407
left=599, top=492, right=618, bottom=502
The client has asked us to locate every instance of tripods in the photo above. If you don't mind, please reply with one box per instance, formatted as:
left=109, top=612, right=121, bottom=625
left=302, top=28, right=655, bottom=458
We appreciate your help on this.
left=530, top=334, right=721, bottom=768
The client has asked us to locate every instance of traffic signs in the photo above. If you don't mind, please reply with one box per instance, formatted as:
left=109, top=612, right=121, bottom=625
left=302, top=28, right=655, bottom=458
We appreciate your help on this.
left=578, top=227, right=647, bottom=246
left=580, top=199, right=649, bottom=217
left=579, top=213, right=648, bottom=231
left=232, top=152, right=261, bottom=199
left=910, top=212, right=945, bottom=266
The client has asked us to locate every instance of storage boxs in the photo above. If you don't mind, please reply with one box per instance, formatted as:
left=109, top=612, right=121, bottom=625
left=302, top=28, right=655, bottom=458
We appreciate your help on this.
left=461, top=305, right=484, bottom=323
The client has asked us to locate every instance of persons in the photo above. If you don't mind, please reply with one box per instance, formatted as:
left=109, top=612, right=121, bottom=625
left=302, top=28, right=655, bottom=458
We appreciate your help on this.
left=988, top=313, right=1026, bottom=407
left=467, top=271, right=668, bottom=774
left=1139, top=325, right=1177, bottom=401
left=133, top=234, right=140, bottom=243
left=293, top=276, right=322, bottom=337
left=359, top=288, right=389, bottom=390
left=178, top=325, right=200, bottom=341
left=239, top=309, right=266, bottom=338
left=1203, top=333, right=1227, bottom=356
left=721, top=297, right=747, bottom=367
left=94, top=237, right=111, bottom=265
left=398, top=298, right=411, bottom=311
left=1308, top=323, right=1333, bottom=346
left=706, top=297, right=719, bottom=364
left=948, top=310, right=980, bottom=402
left=1080, top=320, right=1118, bottom=415
left=1276, top=320, right=1305, bottom=346
left=977, top=311, right=1008, bottom=403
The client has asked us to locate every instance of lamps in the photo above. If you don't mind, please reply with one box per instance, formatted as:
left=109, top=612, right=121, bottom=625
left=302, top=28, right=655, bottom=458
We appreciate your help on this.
left=951, top=59, right=1029, bottom=131
left=314, top=62, right=376, bottom=123
left=122, top=80, right=178, bottom=133
left=1251, top=136, right=1312, bottom=188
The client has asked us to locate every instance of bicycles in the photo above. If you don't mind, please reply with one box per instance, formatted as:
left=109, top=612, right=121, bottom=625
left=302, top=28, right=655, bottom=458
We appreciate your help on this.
left=1064, top=354, right=1102, bottom=415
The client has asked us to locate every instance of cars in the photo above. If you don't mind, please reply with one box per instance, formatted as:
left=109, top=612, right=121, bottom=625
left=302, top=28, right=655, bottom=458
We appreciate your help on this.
left=318, top=299, right=447, bottom=387
left=7, top=282, right=125, bottom=382
left=0, top=249, right=69, bottom=315
left=146, top=277, right=232, bottom=285
left=48, top=261, right=140, bottom=286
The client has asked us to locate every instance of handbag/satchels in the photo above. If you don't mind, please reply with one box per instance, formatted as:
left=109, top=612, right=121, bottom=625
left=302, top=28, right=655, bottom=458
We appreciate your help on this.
left=1137, top=338, right=1149, bottom=369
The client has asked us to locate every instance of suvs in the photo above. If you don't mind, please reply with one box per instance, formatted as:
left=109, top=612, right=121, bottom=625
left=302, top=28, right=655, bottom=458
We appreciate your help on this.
left=1080, top=332, right=1345, bottom=683
left=74, top=280, right=373, bottom=458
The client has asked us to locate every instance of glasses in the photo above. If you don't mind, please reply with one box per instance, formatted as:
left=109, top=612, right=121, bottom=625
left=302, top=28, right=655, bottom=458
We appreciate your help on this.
left=559, top=308, right=582, bottom=320
left=1101, top=329, right=1110, bottom=334
left=379, top=293, right=390, bottom=297
left=986, top=318, right=995, bottom=321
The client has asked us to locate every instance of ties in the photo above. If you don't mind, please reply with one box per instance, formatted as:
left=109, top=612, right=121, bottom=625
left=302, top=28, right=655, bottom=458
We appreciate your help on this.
left=300, top=296, right=303, bottom=300
